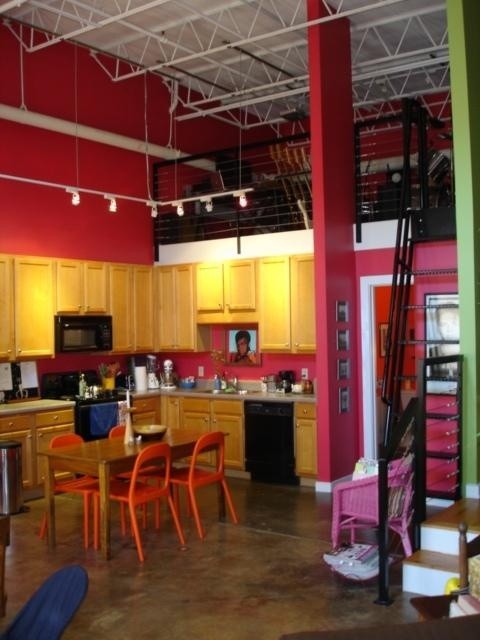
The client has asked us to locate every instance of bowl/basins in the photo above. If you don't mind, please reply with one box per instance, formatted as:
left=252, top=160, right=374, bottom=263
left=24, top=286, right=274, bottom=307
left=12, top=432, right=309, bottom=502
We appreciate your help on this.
left=105, top=390, right=116, bottom=396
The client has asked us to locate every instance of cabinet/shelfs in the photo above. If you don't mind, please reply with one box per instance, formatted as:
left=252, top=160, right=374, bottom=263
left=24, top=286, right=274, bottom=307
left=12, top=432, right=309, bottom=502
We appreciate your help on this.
left=56, top=257, right=111, bottom=316
left=0, top=254, right=58, bottom=361
left=153, top=262, right=212, bottom=353
left=0, top=405, right=77, bottom=495
left=257, top=253, right=317, bottom=355
left=293, top=399, right=317, bottom=487
left=163, top=395, right=179, bottom=436
left=130, top=390, right=162, bottom=441
left=180, top=393, right=246, bottom=473
left=110, top=262, right=157, bottom=353
left=195, top=258, right=257, bottom=324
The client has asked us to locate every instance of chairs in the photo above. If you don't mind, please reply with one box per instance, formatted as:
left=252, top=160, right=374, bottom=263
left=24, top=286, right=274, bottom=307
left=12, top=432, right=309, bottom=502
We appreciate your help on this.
left=109, top=422, right=172, bottom=529
left=329, top=460, right=419, bottom=559
left=93, top=441, right=186, bottom=563
left=0, top=564, right=91, bottom=639
left=154, top=430, right=240, bottom=537
left=39, top=433, right=100, bottom=548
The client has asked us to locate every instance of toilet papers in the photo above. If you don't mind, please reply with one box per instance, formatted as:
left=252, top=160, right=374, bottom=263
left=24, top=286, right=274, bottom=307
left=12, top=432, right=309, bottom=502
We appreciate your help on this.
left=133, top=365, right=148, bottom=392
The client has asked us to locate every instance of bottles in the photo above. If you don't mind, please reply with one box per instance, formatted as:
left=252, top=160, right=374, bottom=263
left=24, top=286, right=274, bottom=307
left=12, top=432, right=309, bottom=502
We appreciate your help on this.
left=303, top=380, right=313, bottom=394
left=80, top=374, right=86, bottom=399
left=213, top=374, right=227, bottom=391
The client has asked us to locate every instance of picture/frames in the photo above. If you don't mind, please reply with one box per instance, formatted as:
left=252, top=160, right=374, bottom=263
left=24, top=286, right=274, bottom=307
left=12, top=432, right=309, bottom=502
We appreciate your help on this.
left=336, top=357, right=350, bottom=381
left=378, top=322, right=392, bottom=358
left=337, top=386, right=354, bottom=414
left=425, top=291, right=458, bottom=398
left=335, top=299, right=350, bottom=323
left=222, top=327, right=262, bottom=368
left=336, top=329, right=350, bottom=350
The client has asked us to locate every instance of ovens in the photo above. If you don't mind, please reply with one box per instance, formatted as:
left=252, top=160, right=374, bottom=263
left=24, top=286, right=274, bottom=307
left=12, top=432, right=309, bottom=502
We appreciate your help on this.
left=76, top=399, right=135, bottom=439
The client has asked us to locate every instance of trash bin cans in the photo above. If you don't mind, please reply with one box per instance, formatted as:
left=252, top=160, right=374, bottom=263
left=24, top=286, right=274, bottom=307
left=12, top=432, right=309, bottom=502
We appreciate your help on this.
left=0, top=440, right=30, bottom=517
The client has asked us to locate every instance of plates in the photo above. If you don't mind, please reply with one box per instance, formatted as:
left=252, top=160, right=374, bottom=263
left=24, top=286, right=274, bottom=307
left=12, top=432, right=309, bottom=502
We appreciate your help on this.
left=178, top=382, right=195, bottom=388
left=132, top=425, right=167, bottom=436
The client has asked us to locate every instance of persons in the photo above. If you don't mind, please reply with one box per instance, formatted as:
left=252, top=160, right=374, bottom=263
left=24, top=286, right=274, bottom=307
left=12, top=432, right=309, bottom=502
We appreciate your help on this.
left=231, top=331, right=256, bottom=365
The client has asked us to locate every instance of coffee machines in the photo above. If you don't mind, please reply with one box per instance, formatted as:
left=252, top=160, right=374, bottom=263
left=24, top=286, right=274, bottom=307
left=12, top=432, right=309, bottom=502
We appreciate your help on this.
left=160, top=361, right=177, bottom=388
left=279, top=370, right=294, bottom=392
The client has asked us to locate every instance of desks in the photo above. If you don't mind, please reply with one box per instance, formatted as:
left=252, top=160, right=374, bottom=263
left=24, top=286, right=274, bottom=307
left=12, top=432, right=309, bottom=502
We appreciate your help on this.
left=38, top=430, right=231, bottom=561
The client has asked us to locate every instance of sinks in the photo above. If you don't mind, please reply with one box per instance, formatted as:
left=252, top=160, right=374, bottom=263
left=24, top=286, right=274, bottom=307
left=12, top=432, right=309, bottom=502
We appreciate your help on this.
left=196, top=388, right=248, bottom=395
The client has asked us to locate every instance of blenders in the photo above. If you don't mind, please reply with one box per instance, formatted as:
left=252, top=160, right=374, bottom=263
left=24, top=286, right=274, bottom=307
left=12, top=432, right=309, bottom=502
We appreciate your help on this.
left=144, top=356, right=159, bottom=389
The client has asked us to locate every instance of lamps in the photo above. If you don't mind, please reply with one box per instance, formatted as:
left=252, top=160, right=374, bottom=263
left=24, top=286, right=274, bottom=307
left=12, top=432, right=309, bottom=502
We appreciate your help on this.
left=1, top=173, right=255, bottom=223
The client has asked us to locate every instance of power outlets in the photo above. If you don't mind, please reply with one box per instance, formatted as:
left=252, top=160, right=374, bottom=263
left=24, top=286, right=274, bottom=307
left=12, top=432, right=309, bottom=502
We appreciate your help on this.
left=301, top=368, right=308, bottom=380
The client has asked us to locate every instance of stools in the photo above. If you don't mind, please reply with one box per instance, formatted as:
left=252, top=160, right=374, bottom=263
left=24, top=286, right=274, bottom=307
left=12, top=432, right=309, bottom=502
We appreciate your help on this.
left=410, top=593, right=463, bottom=624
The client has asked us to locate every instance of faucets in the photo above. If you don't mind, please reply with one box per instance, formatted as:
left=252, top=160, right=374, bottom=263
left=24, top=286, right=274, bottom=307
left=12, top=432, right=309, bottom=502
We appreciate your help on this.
left=233, top=376, right=240, bottom=388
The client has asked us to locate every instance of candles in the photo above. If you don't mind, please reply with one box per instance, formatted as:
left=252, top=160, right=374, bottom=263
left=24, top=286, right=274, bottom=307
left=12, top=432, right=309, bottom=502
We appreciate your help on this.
left=126, top=390, right=131, bottom=409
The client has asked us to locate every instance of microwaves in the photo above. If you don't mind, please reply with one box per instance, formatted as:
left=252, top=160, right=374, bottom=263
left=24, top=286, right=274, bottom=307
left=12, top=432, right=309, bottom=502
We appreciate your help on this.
left=55, top=314, right=114, bottom=352
left=45, top=394, right=127, bottom=405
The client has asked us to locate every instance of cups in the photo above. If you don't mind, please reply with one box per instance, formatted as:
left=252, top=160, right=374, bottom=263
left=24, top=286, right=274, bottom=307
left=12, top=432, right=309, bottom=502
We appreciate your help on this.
left=122, top=375, right=134, bottom=391
left=85, top=386, right=104, bottom=398
left=267, top=375, right=276, bottom=393
left=101, top=377, right=115, bottom=394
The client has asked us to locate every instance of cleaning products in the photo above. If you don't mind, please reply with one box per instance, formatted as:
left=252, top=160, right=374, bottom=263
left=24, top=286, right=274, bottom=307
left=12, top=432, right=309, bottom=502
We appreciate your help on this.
left=220, top=375, right=227, bottom=388
left=214, top=373, right=220, bottom=388
left=79, top=373, right=87, bottom=396
